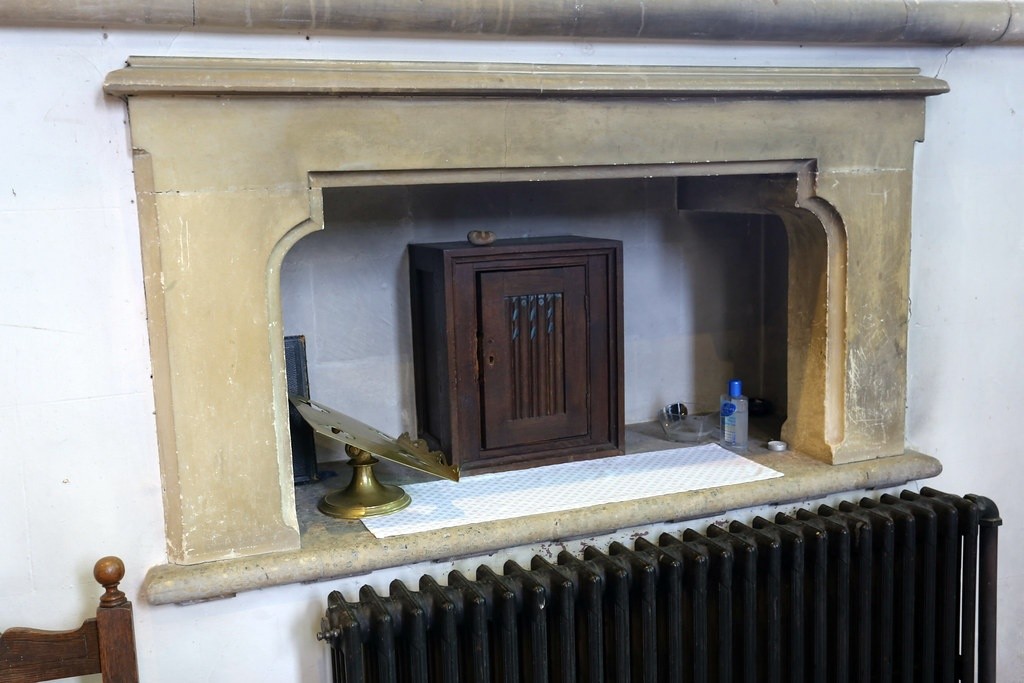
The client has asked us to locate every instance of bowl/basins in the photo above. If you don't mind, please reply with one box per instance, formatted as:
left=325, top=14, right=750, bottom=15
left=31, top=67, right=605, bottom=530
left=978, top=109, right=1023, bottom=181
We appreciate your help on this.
left=658, top=402, right=718, bottom=443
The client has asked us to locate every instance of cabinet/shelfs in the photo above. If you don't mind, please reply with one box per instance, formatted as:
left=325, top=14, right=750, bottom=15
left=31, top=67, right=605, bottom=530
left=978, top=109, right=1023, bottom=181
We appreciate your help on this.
left=407, top=233, right=627, bottom=473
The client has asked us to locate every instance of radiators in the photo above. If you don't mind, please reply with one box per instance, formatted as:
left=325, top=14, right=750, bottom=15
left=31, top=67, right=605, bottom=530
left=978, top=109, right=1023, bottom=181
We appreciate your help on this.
left=316, top=488, right=1002, bottom=683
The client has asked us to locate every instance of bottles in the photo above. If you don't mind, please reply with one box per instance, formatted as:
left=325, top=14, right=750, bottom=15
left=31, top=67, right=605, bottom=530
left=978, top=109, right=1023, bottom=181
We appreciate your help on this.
left=719, top=379, right=749, bottom=447
